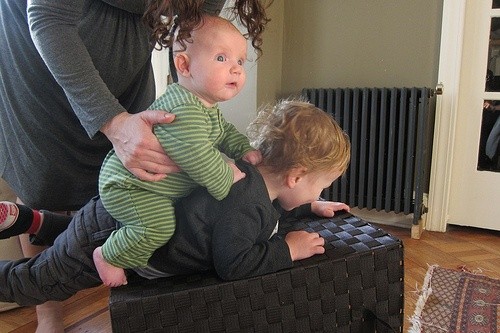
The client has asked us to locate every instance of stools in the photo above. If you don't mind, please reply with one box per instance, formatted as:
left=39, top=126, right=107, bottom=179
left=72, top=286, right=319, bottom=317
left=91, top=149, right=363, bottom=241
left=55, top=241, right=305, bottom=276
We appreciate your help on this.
left=110, top=198, right=405, bottom=333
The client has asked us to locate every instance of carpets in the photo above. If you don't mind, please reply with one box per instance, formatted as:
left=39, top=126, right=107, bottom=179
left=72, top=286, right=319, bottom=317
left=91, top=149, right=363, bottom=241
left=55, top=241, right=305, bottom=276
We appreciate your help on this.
left=408, top=264, right=500, bottom=333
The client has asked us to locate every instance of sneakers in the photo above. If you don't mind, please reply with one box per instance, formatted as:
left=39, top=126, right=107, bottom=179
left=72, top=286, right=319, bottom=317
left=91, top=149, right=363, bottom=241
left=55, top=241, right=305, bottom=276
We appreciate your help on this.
left=0, top=200, right=34, bottom=241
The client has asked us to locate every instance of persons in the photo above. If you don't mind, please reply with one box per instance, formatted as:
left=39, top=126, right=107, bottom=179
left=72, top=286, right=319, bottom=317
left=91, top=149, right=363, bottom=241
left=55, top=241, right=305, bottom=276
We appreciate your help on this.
left=1, top=98, right=351, bottom=309
left=92, top=13, right=263, bottom=287
left=0, top=0, right=275, bottom=333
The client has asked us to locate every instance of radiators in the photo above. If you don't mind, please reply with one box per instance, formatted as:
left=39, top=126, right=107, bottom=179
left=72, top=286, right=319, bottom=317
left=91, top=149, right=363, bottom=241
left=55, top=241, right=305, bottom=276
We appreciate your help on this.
left=302, top=87, right=442, bottom=239
